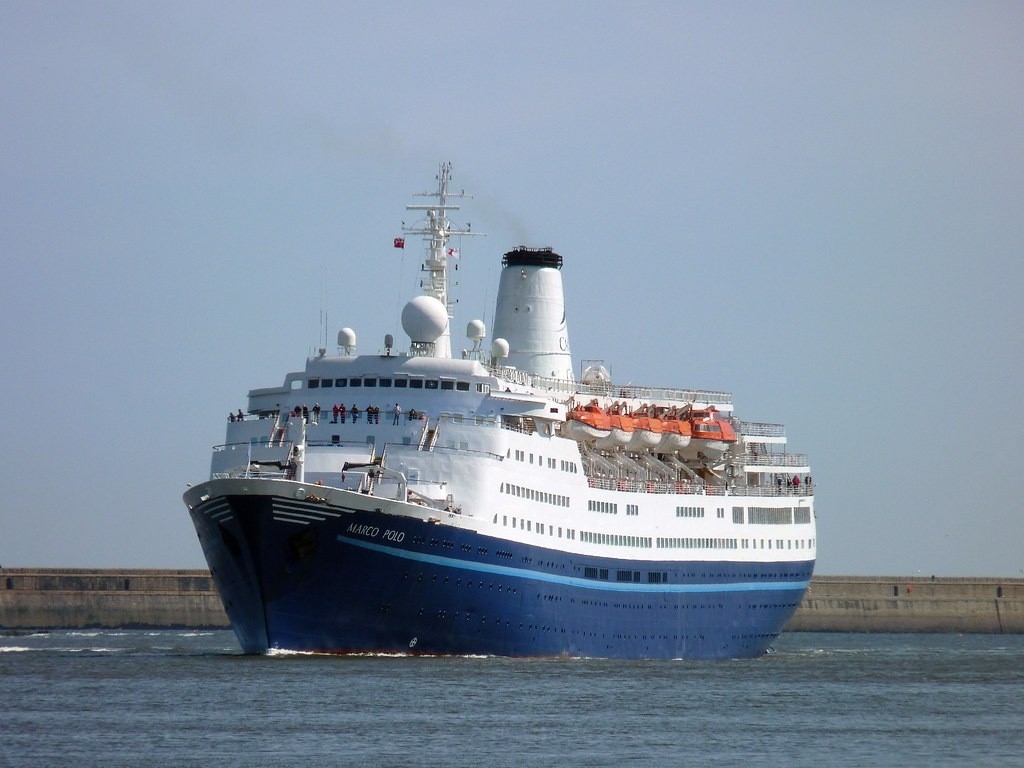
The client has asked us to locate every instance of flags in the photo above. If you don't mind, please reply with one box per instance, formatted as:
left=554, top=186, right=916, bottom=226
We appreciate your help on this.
left=394, top=237, right=404, bottom=248
left=449, top=247, right=460, bottom=259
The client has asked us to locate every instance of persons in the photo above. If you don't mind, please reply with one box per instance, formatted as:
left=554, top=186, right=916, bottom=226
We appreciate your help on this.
left=777, top=479, right=782, bottom=496
left=505, top=387, right=511, bottom=392
left=293, top=402, right=321, bottom=424
left=513, top=422, right=520, bottom=432
left=619, top=388, right=627, bottom=398
left=787, top=475, right=792, bottom=495
left=805, top=473, right=813, bottom=496
left=763, top=452, right=770, bottom=466
left=365, top=469, right=374, bottom=487
left=227, top=412, right=236, bottom=422
left=792, top=475, right=801, bottom=495
left=318, top=479, right=323, bottom=485
left=419, top=413, right=425, bottom=420
left=589, top=473, right=701, bottom=495
left=235, top=409, right=244, bottom=421
left=393, top=403, right=401, bottom=426
left=408, top=409, right=418, bottom=421
left=332, top=403, right=380, bottom=424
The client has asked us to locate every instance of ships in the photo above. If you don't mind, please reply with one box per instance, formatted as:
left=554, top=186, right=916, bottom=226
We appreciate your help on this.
left=178, top=157, right=818, bottom=661
